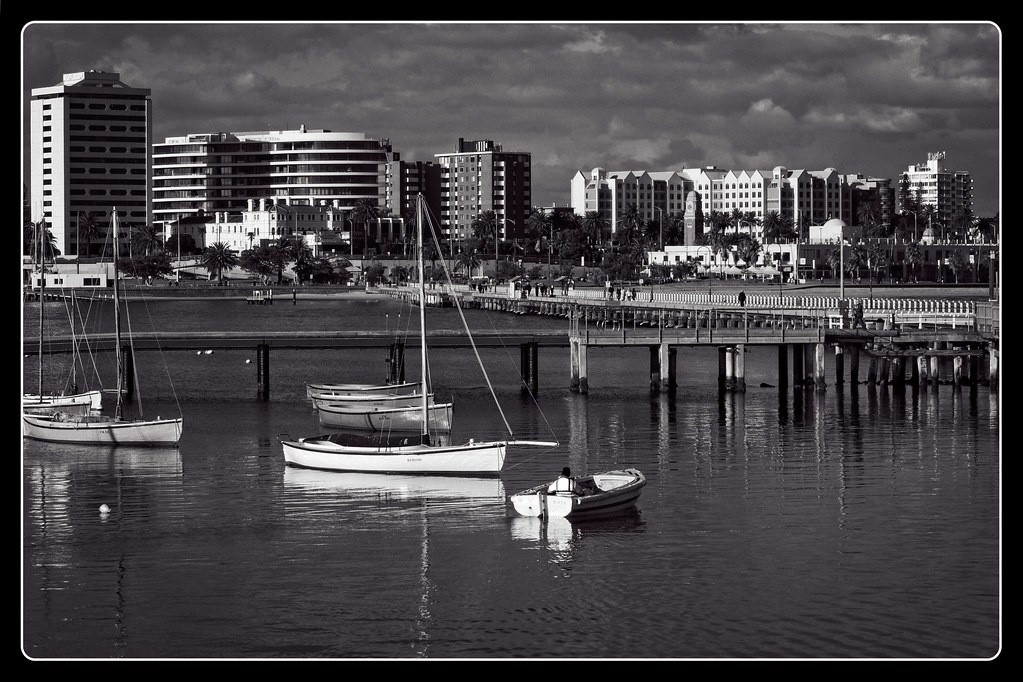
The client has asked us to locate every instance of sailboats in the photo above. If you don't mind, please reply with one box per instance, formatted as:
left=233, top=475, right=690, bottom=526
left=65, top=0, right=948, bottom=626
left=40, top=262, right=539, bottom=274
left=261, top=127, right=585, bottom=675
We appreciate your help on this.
left=23, top=205, right=185, bottom=446
left=278, top=191, right=560, bottom=475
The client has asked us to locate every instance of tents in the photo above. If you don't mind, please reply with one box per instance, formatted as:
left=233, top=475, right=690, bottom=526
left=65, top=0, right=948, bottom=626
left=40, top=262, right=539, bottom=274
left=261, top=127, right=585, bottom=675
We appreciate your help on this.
left=695, top=263, right=782, bottom=282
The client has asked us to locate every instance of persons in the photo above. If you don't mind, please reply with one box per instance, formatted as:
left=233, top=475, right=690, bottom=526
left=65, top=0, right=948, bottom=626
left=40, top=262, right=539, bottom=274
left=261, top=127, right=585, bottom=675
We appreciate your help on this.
left=789, top=269, right=950, bottom=287
left=548, top=467, right=585, bottom=497
left=738, top=289, right=746, bottom=307
left=850, top=299, right=867, bottom=330
left=426, top=277, right=638, bottom=302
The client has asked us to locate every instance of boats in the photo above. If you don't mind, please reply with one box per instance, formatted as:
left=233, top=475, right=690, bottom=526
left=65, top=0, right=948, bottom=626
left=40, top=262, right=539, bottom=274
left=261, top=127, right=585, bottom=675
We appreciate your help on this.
left=509, top=466, right=647, bottom=519
